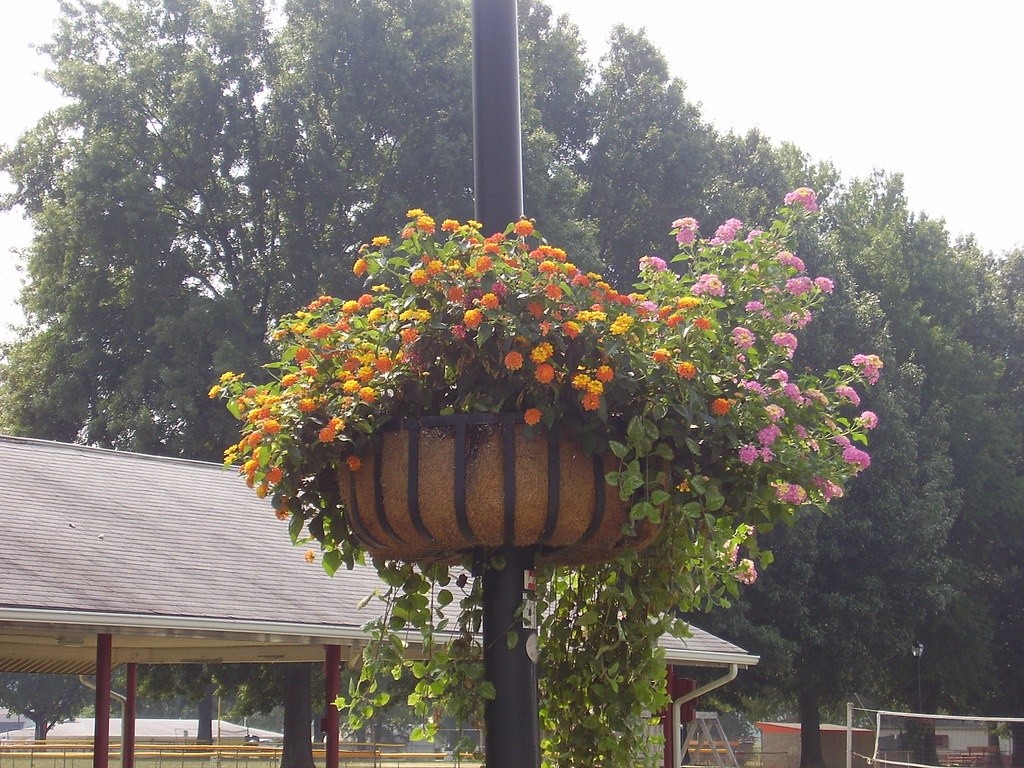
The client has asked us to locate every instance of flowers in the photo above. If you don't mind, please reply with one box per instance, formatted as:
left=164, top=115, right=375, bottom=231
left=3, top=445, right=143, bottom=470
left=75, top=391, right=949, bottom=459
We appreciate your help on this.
left=209, top=185, right=885, bottom=768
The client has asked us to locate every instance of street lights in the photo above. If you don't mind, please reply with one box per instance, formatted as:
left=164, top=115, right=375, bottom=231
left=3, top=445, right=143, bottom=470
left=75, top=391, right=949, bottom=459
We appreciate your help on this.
left=911, top=638, right=925, bottom=763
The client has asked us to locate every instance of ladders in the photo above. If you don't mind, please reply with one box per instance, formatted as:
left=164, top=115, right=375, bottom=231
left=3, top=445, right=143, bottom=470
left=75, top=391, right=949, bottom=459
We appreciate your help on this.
left=681, top=711, right=740, bottom=768
